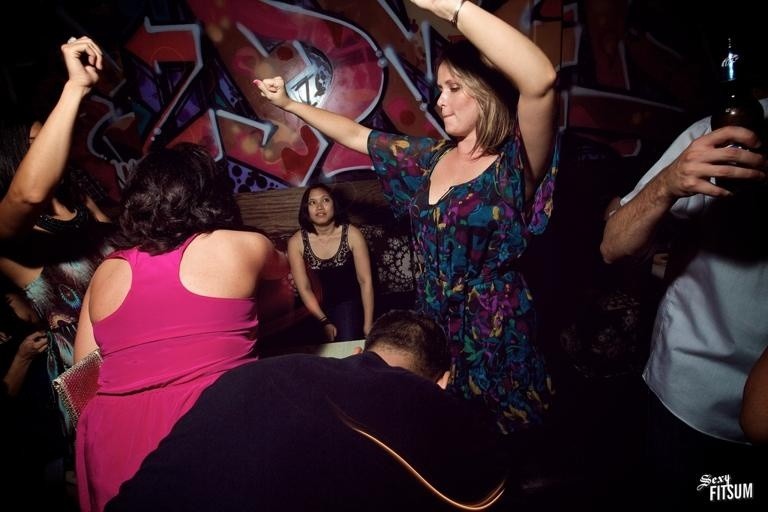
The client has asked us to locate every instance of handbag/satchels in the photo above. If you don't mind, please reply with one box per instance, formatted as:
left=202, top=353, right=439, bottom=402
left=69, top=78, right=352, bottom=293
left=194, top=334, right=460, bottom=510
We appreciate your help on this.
left=52, top=346, right=103, bottom=427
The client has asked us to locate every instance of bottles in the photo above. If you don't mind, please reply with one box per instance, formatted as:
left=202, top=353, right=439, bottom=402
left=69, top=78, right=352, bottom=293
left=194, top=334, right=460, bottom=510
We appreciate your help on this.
left=711, top=36, right=764, bottom=206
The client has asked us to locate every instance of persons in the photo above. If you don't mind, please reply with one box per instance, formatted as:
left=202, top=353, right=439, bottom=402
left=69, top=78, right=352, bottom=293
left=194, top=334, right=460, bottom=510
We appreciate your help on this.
left=1, top=0, right=767, bottom=512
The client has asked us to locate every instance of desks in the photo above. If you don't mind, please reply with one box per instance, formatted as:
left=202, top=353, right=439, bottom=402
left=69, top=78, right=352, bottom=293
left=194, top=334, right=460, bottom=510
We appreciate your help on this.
left=258, top=334, right=367, bottom=364
